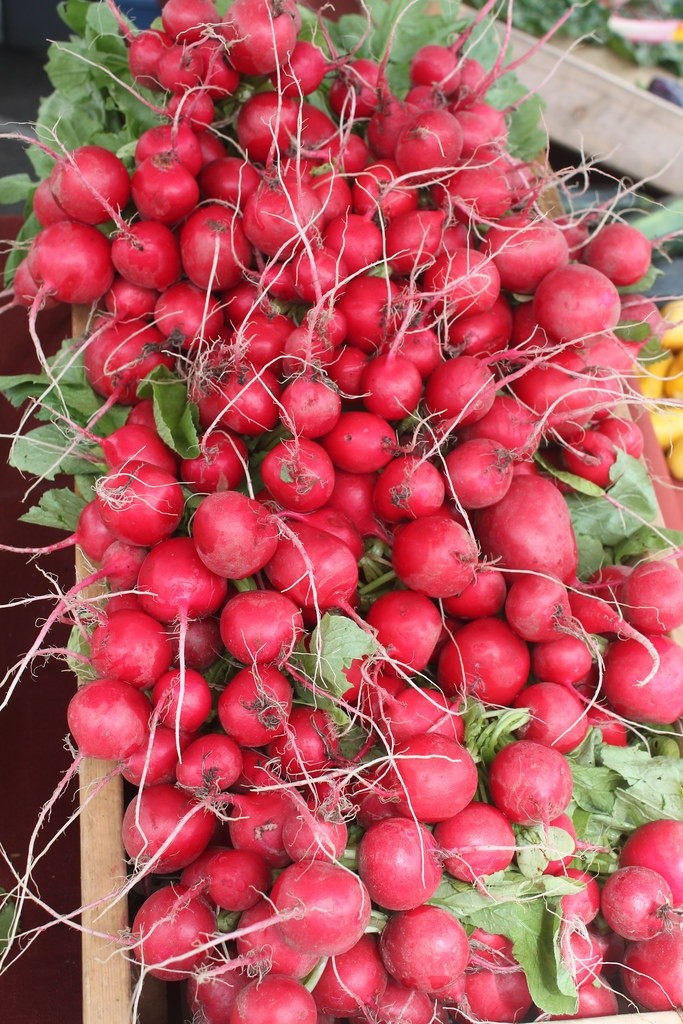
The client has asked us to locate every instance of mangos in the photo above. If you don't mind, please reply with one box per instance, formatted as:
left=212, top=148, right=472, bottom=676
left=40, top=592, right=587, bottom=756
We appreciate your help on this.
left=635, top=301, right=683, bottom=481
